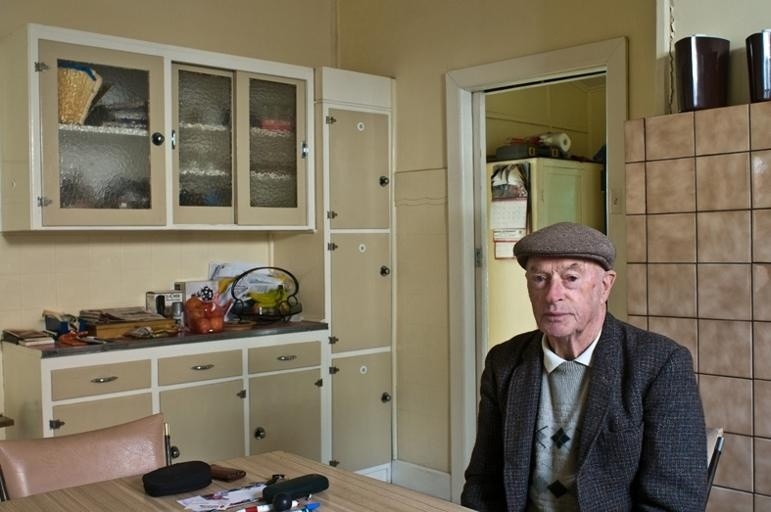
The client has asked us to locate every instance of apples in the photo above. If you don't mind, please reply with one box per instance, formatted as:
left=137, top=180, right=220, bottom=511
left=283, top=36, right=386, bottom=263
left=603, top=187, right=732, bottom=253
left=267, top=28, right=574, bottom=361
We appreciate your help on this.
left=187, top=299, right=223, bottom=334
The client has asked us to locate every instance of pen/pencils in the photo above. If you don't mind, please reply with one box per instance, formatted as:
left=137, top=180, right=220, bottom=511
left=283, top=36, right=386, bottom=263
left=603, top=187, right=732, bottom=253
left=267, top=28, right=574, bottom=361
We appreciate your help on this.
left=237, top=499, right=320, bottom=512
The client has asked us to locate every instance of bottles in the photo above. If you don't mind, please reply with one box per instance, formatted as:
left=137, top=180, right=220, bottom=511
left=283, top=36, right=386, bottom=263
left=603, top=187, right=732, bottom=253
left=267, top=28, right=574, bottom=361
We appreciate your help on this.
left=170, top=301, right=185, bottom=331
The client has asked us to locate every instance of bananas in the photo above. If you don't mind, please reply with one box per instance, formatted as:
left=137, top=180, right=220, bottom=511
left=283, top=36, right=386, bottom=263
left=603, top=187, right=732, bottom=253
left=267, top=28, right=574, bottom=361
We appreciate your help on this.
left=249, top=284, right=289, bottom=308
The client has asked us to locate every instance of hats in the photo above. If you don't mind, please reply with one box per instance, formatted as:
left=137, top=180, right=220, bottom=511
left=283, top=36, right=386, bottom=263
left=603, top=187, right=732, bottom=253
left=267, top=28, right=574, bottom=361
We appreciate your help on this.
left=512, top=222, right=615, bottom=270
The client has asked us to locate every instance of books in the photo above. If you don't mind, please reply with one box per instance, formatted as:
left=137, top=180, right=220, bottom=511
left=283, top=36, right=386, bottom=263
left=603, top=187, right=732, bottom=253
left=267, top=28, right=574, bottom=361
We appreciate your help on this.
left=2, top=328, right=56, bottom=347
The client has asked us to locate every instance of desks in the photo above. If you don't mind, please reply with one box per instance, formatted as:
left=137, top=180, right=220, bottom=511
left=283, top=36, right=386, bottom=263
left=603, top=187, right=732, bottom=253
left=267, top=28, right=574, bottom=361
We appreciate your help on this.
left=0, top=451, right=479, bottom=512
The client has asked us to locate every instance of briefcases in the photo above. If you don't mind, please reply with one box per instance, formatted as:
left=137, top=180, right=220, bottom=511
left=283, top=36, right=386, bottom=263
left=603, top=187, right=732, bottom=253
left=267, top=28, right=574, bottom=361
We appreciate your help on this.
left=495, top=145, right=564, bottom=160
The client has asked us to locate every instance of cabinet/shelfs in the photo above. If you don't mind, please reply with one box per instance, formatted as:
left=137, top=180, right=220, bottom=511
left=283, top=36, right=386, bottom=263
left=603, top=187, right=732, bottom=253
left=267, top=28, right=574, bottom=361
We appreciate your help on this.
left=0, top=23, right=316, bottom=233
left=486, top=159, right=607, bottom=349
left=1, top=321, right=333, bottom=469
left=270, top=66, right=395, bottom=487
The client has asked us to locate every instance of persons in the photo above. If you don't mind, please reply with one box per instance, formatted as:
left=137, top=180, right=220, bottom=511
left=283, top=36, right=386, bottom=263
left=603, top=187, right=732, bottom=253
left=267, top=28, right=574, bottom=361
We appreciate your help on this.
left=457, top=220, right=712, bottom=512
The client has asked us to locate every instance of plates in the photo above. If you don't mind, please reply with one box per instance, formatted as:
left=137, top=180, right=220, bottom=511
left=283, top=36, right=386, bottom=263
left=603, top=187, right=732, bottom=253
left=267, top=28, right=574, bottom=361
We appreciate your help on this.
left=223, top=320, right=257, bottom=331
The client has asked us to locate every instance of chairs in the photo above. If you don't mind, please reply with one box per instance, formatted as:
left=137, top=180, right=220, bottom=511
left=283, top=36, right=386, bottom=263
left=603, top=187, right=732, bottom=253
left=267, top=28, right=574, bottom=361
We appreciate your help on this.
left=0, top=412, right=171, bottom=502
left=704, top=427, right=725, bottom=512
